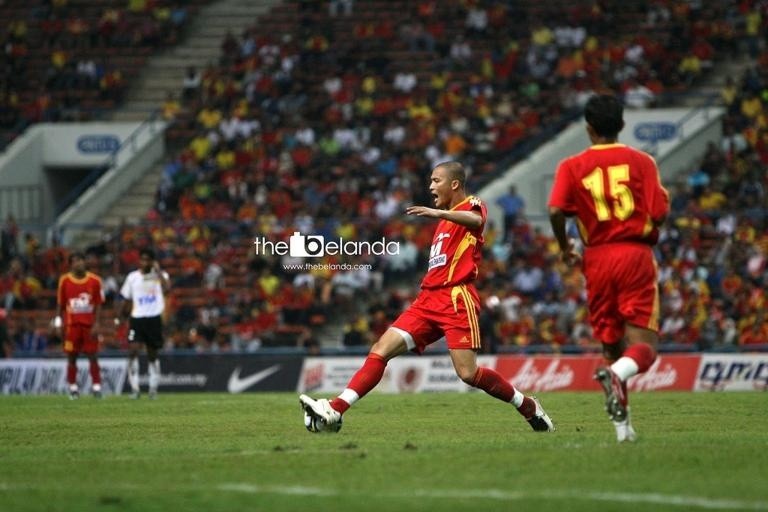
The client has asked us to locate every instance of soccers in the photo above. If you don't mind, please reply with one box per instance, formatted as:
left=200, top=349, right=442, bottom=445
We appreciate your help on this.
left=304, top=399, right=341, bottom=433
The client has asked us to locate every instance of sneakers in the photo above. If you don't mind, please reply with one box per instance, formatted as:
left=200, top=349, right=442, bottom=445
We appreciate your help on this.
left=67, top=389, right=102, bottom=399
left=128, top=390, right=158, bottom=400
left=591, top=365, right=628, bottom=421
left=298, top=393, right=341, bottom=426
left=525, top=394, right=553, bottom=431
left=615, top=432, right=636, bottom=442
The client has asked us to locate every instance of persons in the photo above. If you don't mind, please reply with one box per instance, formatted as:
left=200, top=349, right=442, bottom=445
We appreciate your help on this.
left=113, top=247, right=172, bottom=402
left=52, top=248, right=108, bottom=402
left=547, top=91, right=670, bottom=445
left=0, top=1, right=768, bottom=357
left=296, top=162, right=555, bottom=432
